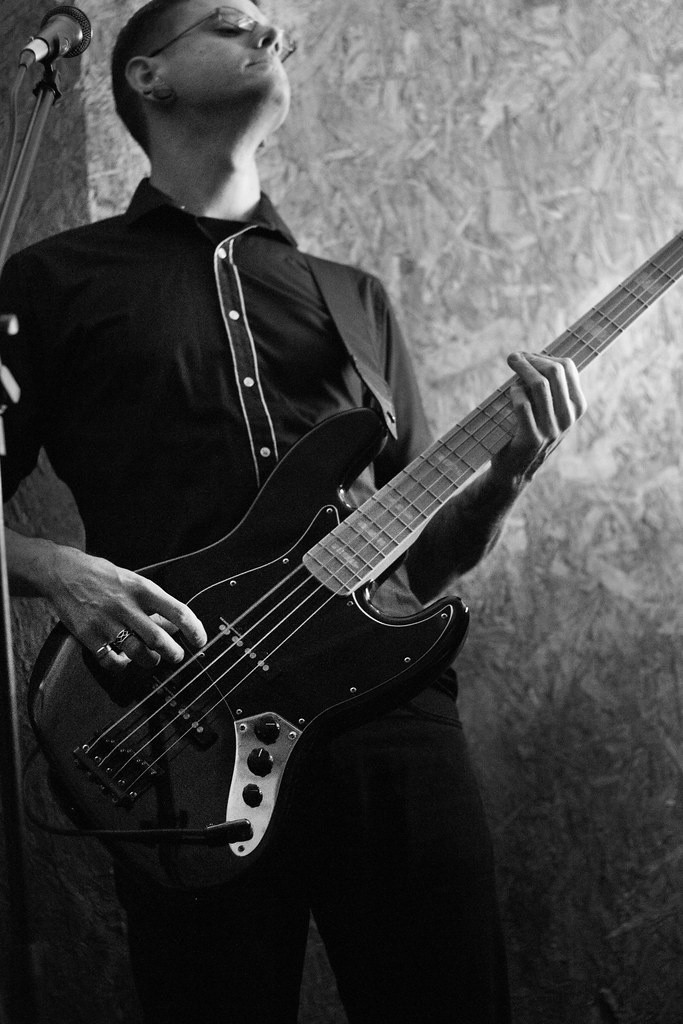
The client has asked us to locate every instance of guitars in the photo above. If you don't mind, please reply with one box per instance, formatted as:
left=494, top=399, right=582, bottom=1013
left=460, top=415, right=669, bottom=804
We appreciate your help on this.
left=29, top=229, right=682, bottom=919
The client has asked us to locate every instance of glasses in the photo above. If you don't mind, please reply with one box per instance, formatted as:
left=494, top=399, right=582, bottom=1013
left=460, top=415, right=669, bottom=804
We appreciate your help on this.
left=145, top=5, right=297, bottom=63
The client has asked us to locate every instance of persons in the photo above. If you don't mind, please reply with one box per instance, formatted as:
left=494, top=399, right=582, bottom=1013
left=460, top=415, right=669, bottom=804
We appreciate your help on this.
left=0, top=0, right=587, bottom=1023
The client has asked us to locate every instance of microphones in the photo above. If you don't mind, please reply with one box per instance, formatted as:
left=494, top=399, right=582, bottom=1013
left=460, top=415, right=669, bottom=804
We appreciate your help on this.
left=205, top=819, right=254, bottom=849
left=20, top=6, right=93, bottom=73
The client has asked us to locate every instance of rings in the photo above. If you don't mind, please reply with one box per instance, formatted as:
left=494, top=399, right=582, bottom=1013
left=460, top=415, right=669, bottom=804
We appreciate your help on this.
left=95, top=643, right=112, bottom=659
left=112, top=628, right=134, bottom=649
